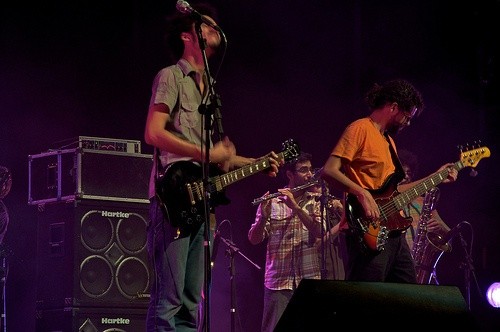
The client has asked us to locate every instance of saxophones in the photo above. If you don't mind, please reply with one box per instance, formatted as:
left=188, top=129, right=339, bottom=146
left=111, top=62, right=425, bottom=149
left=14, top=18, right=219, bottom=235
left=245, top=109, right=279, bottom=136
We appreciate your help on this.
left=411, top=186, right=453, bottom=285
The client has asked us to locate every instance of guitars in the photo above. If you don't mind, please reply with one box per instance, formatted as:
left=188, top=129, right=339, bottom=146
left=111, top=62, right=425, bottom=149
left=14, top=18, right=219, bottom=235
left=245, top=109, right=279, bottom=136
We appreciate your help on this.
left=154, top=138, right=302, bottom=238
left=344, top=140, right=491, bottom=256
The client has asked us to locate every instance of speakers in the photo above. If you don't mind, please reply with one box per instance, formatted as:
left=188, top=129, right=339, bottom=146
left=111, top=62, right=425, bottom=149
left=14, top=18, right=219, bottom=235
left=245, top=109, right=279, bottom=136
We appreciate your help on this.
left=274, top=279, right=471, bottom=332
left=33, top=199, right=155, bottom=332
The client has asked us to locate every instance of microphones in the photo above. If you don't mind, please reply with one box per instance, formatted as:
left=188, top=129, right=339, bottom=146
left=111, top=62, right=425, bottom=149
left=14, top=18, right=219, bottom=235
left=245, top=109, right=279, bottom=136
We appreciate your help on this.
left=175, top=0, right=222, bottom=31
left=212, top=226, right=223, bottom=262
left=440, top=224, right=461, bottom=245
left=312, top=168, right=322, bottom=180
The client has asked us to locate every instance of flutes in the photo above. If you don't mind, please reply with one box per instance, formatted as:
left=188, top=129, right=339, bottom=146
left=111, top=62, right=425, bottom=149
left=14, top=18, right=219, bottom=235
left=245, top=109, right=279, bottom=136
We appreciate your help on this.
left=250, top=180, right=319, bottom=206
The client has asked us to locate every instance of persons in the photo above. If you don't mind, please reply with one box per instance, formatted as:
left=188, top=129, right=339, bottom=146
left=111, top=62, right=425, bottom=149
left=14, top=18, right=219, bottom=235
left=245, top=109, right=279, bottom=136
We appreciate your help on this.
left=144, top=16, right=279, bottom=332
left=248, top=152, right=348, bottom=332
left=320, top=78, right=458, bottom=284
left=391, top=146, right=443, bottom=256
